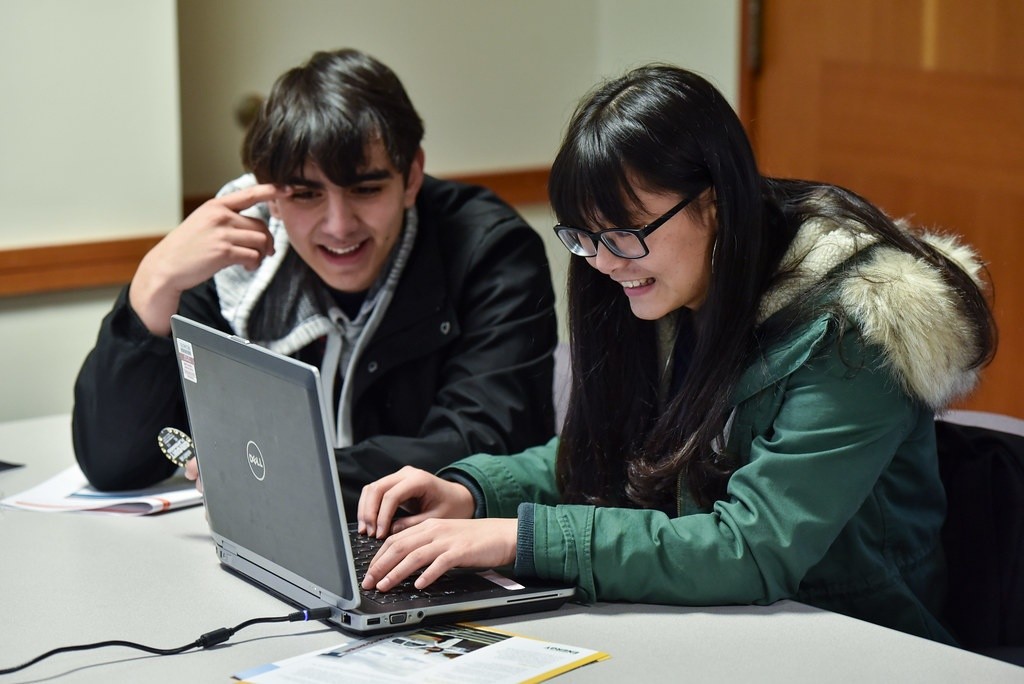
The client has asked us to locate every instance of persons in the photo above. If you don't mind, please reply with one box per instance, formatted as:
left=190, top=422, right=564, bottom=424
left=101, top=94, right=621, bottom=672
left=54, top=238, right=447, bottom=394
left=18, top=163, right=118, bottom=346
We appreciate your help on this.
left=71, top=48, right=559, bottom=523
left=357, top=63, right=1000, bottom=649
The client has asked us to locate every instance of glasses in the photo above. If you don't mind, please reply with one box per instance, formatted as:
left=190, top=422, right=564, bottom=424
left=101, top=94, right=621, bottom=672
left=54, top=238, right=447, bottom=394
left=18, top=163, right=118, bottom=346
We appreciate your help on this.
left=553, top=182, right=713, bottom=259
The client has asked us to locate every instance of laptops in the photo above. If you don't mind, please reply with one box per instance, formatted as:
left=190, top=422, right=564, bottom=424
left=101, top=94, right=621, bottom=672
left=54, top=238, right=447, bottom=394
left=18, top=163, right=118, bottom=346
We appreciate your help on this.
left=169, top=313, right=576, bottom=638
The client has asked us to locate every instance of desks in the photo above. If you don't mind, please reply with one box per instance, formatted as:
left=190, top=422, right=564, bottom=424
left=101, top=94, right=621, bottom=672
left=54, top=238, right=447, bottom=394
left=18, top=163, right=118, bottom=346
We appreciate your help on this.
left=0, top=411, right=1024, bottom=684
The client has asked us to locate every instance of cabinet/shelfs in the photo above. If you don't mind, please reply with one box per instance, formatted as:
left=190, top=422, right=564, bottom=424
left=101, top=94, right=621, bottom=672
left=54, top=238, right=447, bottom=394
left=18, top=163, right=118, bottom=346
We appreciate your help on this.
left=739, top=0, right=1024, bottom=436
